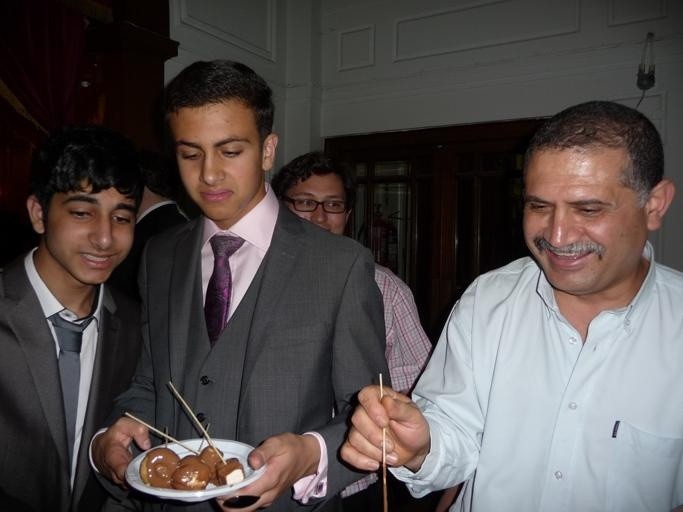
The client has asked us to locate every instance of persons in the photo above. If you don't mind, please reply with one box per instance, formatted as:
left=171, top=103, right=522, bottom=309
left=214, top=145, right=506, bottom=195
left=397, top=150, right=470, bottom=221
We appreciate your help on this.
left=340, top=99, right=683, bottom=512
left=272, top=153, right=464, bottom=512
left=105, top=167, right=189, bottom=312
left=89, top=60, right=390, bottom=512
left=0, top=124, right=148, bottom=507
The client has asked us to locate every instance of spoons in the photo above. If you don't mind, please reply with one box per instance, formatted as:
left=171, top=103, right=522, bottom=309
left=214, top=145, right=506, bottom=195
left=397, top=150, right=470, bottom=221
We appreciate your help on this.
left=222, top=494, right=260, bottom=508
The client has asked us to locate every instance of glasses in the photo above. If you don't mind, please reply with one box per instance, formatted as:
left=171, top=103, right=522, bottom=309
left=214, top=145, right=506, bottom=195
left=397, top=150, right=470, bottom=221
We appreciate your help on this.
left=282, top=194, right=348, bottom=215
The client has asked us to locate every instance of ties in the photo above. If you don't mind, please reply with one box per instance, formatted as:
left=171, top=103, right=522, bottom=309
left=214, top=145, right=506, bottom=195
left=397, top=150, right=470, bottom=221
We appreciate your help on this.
left=201, top=233, right=245, bottom=347
left=49, top=311, right=90, bottom=471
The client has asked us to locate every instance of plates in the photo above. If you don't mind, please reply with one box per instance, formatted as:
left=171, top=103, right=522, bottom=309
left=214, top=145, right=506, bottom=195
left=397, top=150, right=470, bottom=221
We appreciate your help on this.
left=125, top=437, right=266, bottom=502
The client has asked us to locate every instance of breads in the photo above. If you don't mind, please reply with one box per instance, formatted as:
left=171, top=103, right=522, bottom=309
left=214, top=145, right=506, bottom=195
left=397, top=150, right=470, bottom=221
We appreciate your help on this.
left=140, top=444, right=244, bottom=490
